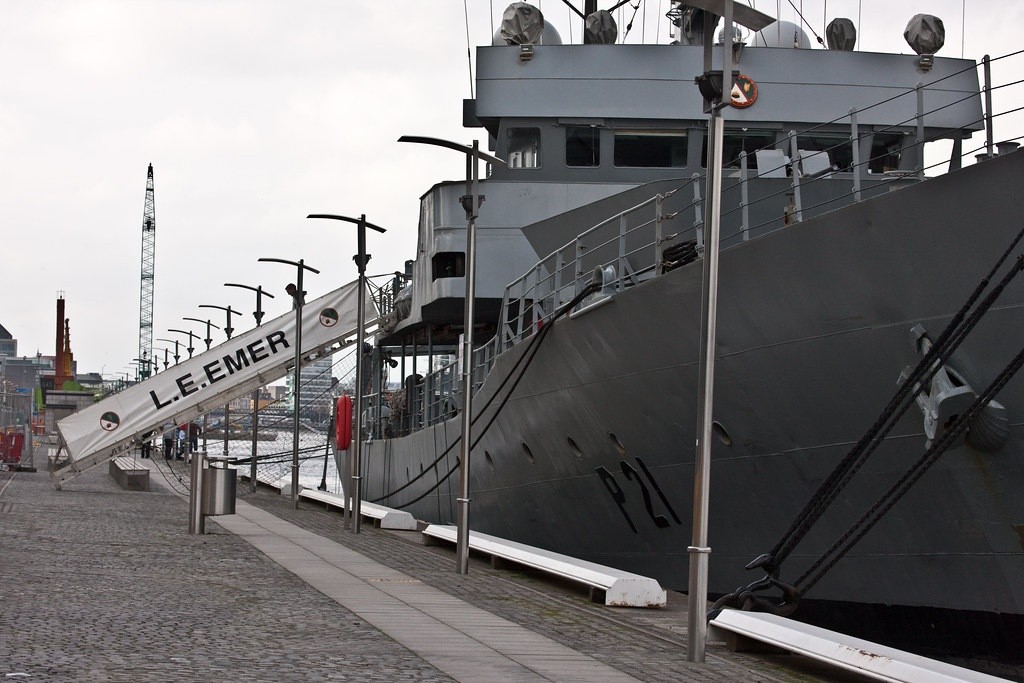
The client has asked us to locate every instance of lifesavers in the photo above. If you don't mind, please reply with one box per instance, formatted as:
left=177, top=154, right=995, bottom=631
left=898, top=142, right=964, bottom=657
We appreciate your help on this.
left=335, top=396, right=353, bottom=450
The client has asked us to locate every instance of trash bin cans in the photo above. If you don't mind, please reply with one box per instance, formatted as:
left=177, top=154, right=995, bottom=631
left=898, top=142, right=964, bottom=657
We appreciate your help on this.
left=189, top=450, right=238, bottom=535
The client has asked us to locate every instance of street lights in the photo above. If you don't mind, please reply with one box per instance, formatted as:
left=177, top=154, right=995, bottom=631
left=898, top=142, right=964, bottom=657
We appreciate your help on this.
left=290, top=213, right=389, bottom=533
left=397, top=136, right=508, bottom=577
left=198, top=304, right=243, bottom=471
left=156, top=338, right=186, bottom=458
left=224, top=282, right=274, bottom=493
left=183, top=317, right=220, bottom=452
left=167, top=329, right=201, bottom=465
left=258, top=258, right=320, bottom=509
left=672, top=0, right=778, bottom=662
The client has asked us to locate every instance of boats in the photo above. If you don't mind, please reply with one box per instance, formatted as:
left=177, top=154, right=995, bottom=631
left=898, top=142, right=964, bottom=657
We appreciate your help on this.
left=326, top=0, right=1024, bottom=682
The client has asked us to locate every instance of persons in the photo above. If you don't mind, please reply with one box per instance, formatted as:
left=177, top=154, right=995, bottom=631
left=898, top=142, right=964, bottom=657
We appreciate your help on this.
left=161, top=418, right=202, bottom=462
left=141, top=430, right=152, bottom=458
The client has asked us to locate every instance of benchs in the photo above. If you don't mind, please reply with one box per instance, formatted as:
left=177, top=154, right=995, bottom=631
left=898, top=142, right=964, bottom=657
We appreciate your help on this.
left=110, top=456, right=151, bottom=490
left=47, top=448, right=69, bottom=469
